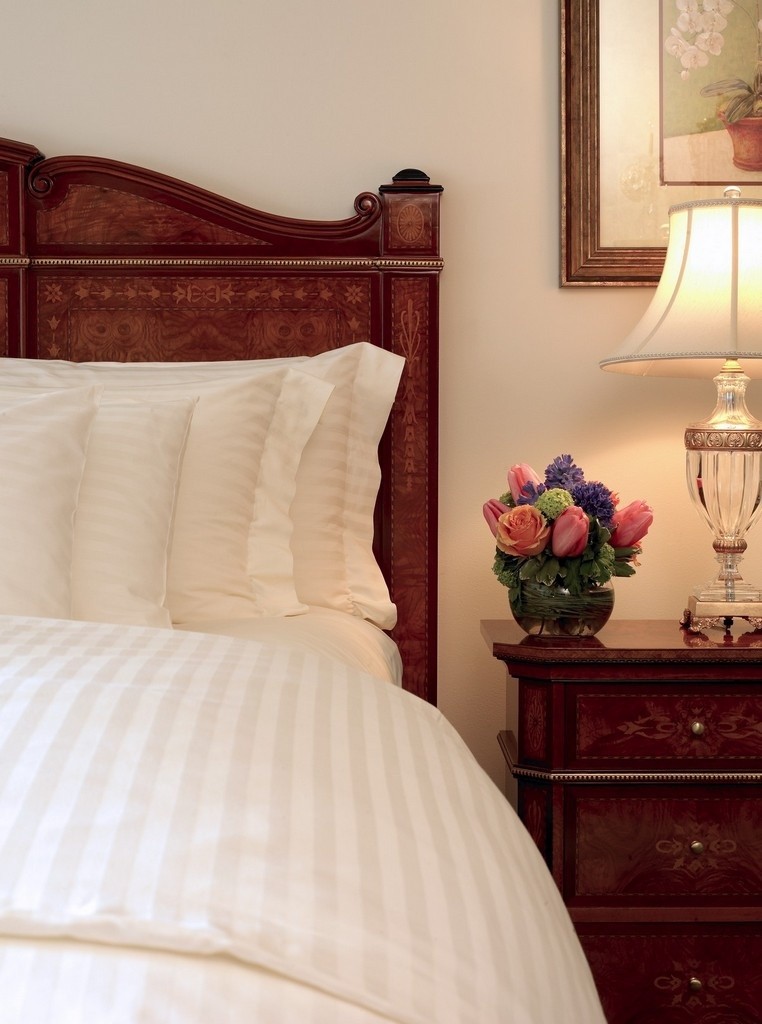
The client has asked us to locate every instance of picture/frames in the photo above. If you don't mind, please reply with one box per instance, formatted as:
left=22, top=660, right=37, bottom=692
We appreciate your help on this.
left=559, top=0, right=762, bottom=288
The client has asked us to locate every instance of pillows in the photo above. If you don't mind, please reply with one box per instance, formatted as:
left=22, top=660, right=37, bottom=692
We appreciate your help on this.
left=0, top=341, right=398, bottom=632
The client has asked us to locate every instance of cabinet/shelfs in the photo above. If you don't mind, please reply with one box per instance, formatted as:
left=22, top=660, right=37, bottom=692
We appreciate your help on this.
left=479, top=619, right=762, bottom=1024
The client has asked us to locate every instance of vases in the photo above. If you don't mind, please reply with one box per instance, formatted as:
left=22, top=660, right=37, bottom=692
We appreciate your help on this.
left=509, top=580, right=615, bottom=639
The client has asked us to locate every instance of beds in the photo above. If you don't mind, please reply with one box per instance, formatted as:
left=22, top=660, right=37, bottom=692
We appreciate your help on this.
left=0, top=137, right=444, bottom=1024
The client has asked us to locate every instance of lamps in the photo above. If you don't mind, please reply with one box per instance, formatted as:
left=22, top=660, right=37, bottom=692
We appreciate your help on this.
left=599, top=186, right=762, bottom=633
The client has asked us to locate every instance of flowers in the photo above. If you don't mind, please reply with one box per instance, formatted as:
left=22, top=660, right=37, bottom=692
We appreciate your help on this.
left=483, top=454, right=653, bottom=632
